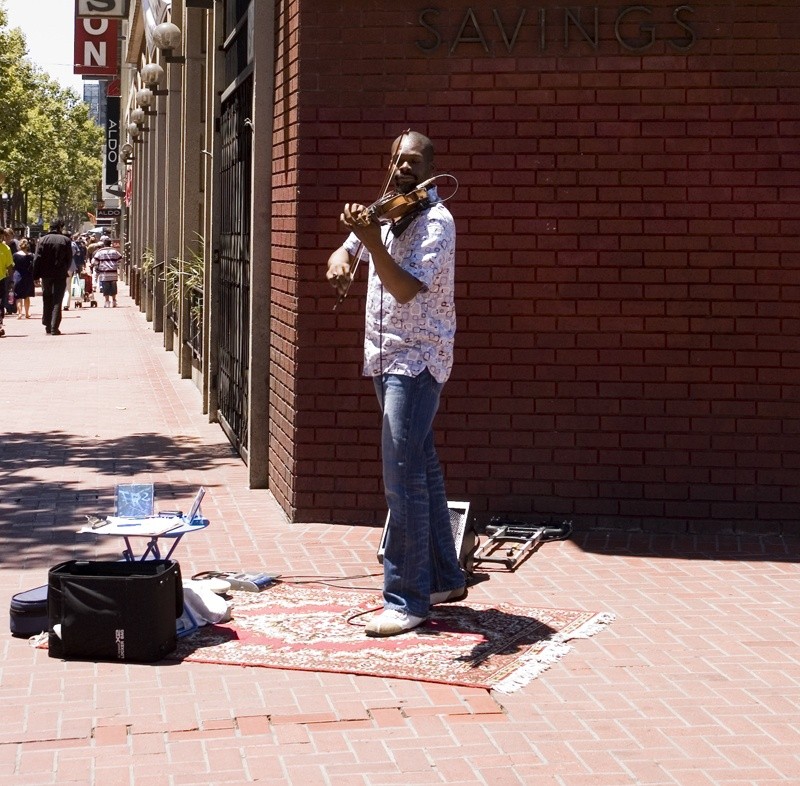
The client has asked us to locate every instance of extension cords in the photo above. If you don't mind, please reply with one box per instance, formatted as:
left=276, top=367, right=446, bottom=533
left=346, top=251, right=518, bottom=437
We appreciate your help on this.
left=209, top=571, right=274, bottom=592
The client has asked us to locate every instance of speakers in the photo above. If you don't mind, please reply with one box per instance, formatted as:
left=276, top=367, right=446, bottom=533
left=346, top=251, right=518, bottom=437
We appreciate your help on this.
left=377, top=500, right=475, bottom=573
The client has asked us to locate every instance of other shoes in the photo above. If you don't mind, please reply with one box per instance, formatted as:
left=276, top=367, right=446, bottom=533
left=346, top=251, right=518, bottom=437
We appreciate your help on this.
left=112, top=300, right=117, bottom=307
left=46, top=328, right=62, bottom=335
left=104, top=301, right=110, bottom=307
left=16, top=314, right=22, bottom=320
left=64, top=306, right=69, bottom=311
left=25, top=314, right=32, bottom=318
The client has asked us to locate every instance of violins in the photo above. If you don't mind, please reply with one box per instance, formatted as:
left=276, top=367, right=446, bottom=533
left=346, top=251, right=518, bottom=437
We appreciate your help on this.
left=356, top=187, right=429, bottom=227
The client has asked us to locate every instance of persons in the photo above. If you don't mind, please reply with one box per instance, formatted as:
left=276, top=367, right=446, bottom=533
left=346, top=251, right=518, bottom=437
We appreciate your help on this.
left=0, top=227, right=113, bottom=338
left=91, top=238, right=123, bottom=308
left=32, top=218, right=72, bottom=335
left=326, top=132, right=466, bottom=637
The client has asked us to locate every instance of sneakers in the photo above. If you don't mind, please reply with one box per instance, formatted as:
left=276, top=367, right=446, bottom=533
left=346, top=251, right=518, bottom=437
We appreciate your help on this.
left=364, top=610, right=427, bottom=636
left=429, top=585, right=465, bottom=605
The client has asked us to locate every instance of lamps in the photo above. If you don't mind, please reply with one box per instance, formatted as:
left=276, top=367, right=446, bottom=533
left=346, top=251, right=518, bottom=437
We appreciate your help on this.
left=120, top=153, right=132, bottom=165
left=127, top=123, right=143, bottom=143
left=131, top=108, right=149, bottom=132
left=141, top=64, right=168, bottom=96
left=121, top=143, right=135, bottom=160
left=152, top=22, right=185, bottom=63
left=136, top=88, right=157, bottom=115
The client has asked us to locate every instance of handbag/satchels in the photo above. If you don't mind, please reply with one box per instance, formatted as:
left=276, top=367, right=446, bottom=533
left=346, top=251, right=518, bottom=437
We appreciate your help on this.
left=71, top=273, right=86, bottom=298
left=48, top=558, right=184, bottom=662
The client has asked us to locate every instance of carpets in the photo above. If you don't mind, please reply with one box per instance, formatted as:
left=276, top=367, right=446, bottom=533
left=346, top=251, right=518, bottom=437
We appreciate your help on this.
left=164, top=584, right=617, bottom=696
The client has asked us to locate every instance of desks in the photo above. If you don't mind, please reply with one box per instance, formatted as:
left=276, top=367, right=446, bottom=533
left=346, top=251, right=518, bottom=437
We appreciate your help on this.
left=81, top=515, right=210, bottom=563
